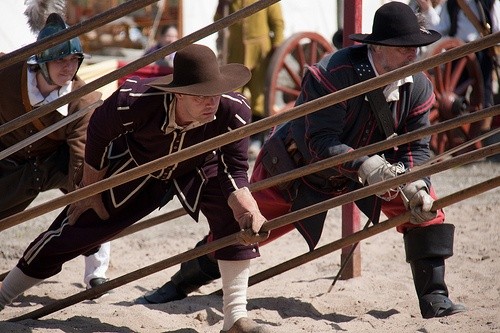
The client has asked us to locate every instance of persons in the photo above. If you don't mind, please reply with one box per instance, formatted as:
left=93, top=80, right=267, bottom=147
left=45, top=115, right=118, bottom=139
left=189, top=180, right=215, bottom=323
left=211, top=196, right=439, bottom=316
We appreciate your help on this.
left=1, top=44, right=269, bottom=333
left=215, top=0, right=286, bottom=155
left=132, top=2, right=464, bottom=318
left=147, top=26, right=179, bottom=60
left=0, top=12, right=113, bottom=294
left=408, top=0, right=500, bottom=104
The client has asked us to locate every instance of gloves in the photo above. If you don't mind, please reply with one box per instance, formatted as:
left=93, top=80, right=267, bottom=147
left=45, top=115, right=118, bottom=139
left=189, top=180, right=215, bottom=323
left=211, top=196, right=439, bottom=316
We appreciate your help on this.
left=410, top=188, right=438, bottom=224
left=367, top=165, right=406, bottom=196
left=67, top=159, right=112, bottom=225
left=228, top=186, right=272, bottom=247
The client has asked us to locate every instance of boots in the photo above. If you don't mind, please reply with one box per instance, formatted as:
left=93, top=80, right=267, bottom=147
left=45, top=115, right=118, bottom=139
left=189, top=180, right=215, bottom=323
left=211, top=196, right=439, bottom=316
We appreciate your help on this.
left=402, top=222, right=465, bottom=319
left=135, top=254, right=212, bottom=304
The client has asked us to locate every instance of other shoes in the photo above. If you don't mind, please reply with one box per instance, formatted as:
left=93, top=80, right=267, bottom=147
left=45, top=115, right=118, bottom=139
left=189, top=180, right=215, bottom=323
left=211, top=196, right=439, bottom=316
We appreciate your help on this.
left=88, top=277, right=114, bottom=301
left=220, top=316, right=272, bottom=333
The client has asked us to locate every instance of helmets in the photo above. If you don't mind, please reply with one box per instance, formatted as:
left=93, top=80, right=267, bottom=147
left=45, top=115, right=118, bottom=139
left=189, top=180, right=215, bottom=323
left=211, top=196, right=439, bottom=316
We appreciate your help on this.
left=27, top=12, right=92, bottom=65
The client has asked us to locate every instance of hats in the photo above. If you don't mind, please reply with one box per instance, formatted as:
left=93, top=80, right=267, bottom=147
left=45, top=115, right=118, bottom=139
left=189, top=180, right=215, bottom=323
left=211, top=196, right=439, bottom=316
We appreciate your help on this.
left=142, top=44, right=252, bottom=97
left=349, top=1, right=442, bottom=47
